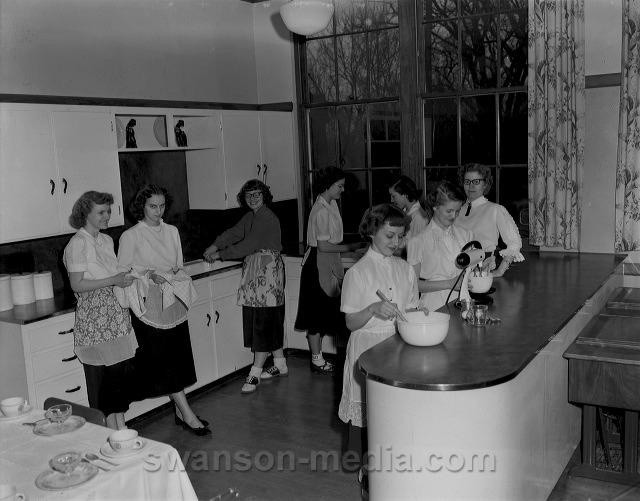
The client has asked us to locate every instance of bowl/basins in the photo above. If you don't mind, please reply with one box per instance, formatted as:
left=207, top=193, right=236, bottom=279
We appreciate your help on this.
left=396, top=312, right=450, bottom=347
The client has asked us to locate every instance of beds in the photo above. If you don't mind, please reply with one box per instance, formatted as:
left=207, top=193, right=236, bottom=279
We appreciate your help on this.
left=0, top=409, right=200, bottom=501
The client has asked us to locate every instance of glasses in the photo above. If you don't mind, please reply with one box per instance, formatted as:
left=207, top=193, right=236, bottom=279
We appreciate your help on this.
left=463, top=178, right=485, bottom=185
left=244, top=192, right=262, bottom=199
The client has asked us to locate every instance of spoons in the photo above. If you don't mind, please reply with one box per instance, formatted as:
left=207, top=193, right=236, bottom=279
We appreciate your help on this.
left=86, top=453, right=118, bottom=467
left=81, top=458, right=110, bottom=473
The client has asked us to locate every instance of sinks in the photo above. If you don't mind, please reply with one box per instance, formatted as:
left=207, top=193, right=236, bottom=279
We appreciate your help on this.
left=183, top=258, right=242, bottom=276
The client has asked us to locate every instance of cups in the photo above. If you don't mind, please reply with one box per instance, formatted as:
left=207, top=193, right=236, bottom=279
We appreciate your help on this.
left=109, top=429, right=142, bottom=452
left=50, top=453, right=82, bottom=474
left=471, top=305, right=488, bottom=328
left=1, top=397, right=27, bottom=417
left=0, top=485, right=25, bottom=501
left=46, top=404, right=72, bottom=423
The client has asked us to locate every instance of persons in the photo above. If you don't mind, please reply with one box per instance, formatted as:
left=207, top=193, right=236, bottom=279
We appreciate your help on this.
left=117, top=184, right=213, bottom=435
left=203, top=179, right=288, bottom=395
left=338, top=163, right=522, bottom=472
left=294, top=164, right=368, bottom=374
left=63, top=190, right=139, bottom=431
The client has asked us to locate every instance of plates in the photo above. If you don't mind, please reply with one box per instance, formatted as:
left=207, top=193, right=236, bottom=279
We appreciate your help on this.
left=0, top=404, right=32, bottom=421
left=116, top=117, right=127, bottom=150
left=100, top=443, right=143, bottom=457
left=33, top=416, right=85, bottom=436
left=35, top=464, right=97, bottom=491
left=153, top=116, right=167, bottom=148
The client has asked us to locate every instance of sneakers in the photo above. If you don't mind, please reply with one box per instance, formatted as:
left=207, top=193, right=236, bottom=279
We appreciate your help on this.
left=242, top=376, right=260, bottom=393
left=261, top=366, right=289, bottom=379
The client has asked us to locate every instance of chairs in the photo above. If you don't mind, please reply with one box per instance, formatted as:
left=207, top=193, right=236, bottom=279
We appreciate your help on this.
left=44, top=396, right=104, bottom=425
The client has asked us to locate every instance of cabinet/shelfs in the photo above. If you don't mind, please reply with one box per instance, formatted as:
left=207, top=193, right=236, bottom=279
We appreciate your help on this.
left=283, top=258, right=336, bottom=356
left=0, top=268, right=284, bottom=422
left=185, top=109, right=297, bottom=210
left=115, top=113, right=219, bottom=152
left=0, top=102, right=125, bottom=244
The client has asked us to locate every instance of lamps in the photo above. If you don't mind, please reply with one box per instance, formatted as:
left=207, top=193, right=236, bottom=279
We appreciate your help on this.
left=279, top=0, right=335, bottom=36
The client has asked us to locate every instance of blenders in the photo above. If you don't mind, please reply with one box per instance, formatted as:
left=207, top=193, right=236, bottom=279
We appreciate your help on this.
left=457, top=240, right=501, bottom=311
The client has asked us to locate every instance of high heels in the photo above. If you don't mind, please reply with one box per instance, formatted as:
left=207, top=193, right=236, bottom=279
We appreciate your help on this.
left=310, top=360, right=337, bottom=377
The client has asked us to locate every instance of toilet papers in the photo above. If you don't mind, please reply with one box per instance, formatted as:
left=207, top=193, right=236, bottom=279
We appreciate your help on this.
left=0, top=274, right=14, bottom=312
left=33, top=271, right=55, bottom=300
left=10, top=273, right=36, bottom=305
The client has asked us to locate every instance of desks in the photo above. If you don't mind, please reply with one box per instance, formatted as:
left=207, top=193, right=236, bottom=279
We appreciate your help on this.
left=562, top=286, right=639, bottom=488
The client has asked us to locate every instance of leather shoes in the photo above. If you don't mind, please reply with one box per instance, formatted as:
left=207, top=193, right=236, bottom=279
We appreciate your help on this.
left=174, top=413, right=212, bottom=436
